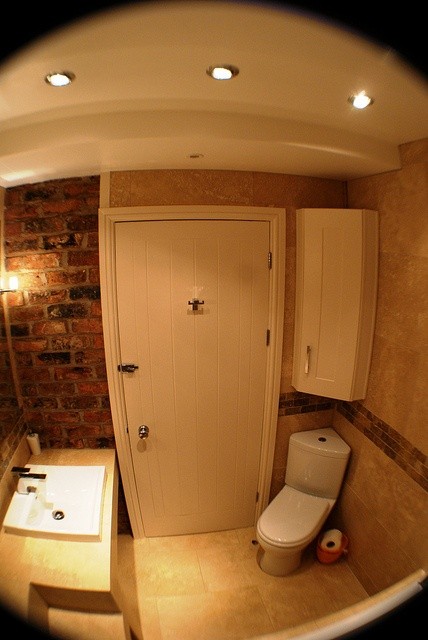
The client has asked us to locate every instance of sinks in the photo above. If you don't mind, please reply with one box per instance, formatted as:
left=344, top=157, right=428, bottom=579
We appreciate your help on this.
left=23, top=468, right=102, bottom=534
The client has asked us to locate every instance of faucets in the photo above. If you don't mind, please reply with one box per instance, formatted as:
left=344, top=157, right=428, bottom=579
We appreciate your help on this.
left=13, top=465, right=48, bottom=494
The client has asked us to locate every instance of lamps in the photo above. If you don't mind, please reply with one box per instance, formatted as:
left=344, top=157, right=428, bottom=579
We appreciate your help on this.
left=0, top=276, right=20, bottom=293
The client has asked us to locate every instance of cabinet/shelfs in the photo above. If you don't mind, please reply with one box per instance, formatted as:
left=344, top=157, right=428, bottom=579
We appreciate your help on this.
left=291, top=208, right=380, bottom=402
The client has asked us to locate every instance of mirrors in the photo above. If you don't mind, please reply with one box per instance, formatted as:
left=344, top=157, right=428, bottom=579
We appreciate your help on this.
left=0, top=304, right=29, bottom=484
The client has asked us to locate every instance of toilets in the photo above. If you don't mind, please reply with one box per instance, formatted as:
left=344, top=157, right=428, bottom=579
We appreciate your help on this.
left=256, top=427, right=351, bottom=576
